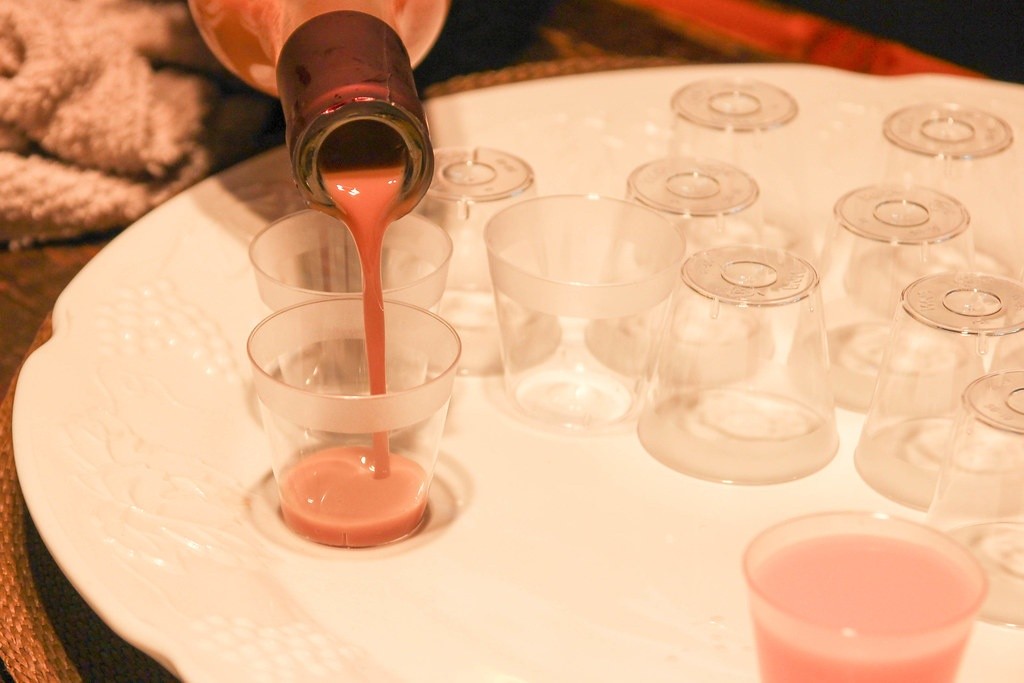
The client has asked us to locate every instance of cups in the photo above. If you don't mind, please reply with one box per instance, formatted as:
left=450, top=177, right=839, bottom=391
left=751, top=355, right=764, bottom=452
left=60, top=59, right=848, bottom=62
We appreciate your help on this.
left=411, top=145, right=561, bottom=374
left=881, top=101, right=1015, bottom=264
left=817, top=184, right=974, bottom=415
left=927, top=368, right=1024, bottom=628
left=246, top=297, right=462, bottom=549
left=744, top=510, right=985, bottom=683
left=854, top=271, right=1024, bottom=510
left=668, top=74, right=806, bottom=256
left=583, top=157, right=765, bottom=377
left=483, top=194, right=685, bottom=432
left=637, top=245, right=840, bottom=486
left=248, top=209, right=453, bottom=320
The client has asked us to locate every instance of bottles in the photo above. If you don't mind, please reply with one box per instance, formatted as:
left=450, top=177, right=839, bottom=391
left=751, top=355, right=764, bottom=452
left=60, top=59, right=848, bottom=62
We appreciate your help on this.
left=183, top=0, right=449, bottom=231
